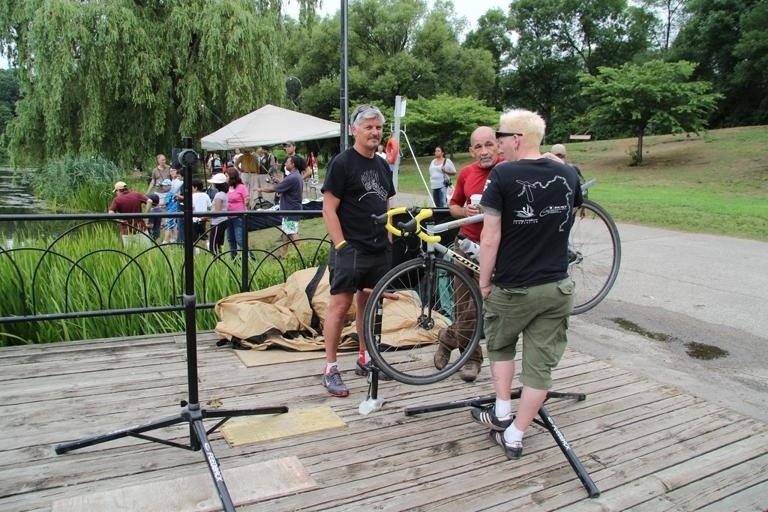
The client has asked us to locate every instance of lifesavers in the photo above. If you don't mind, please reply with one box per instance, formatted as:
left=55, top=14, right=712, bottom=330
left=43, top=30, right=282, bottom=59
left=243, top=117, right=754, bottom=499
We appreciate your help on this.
left=386, top=138, right=398, bottom=164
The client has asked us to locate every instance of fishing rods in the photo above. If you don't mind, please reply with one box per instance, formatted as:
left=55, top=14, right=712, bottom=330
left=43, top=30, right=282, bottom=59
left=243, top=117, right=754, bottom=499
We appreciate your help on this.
left=201, top=104, right=274, bottom=184
left=149, top=153, right=164, bottom=180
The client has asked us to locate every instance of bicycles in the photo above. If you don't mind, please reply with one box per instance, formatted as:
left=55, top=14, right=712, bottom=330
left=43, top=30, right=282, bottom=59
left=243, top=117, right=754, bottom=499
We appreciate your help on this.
left=363, top=179, right=622, bottom=384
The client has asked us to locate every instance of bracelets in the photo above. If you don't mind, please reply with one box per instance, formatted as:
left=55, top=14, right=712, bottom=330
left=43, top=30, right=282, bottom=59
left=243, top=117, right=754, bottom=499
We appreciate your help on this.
left=481, top=284, right=491, bottom=289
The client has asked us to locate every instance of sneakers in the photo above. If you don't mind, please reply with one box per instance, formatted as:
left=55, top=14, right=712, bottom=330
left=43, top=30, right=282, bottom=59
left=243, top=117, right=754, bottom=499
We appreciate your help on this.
left=434, top=327, right=453, bottom=370
left=355, top=360, right=393, bottom=380
left=461, top=360, right=480, bottom=381
left=470, top=405, right=514, bottom=430
left=273, top=255, right=289, bottom=260
left=321, top=365, right=349, bottom=397
left=489, top=429, right=523, bottom=459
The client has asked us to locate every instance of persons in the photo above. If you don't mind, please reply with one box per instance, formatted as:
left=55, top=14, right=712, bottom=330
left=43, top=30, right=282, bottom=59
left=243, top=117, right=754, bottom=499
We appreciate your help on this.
left=434, top=127, right=506, bottom=380
left=375, top=144, right=456, bottom=208
left=108, top=142, right=317, bottom=266
left=319, top=104, right=397, bottom=398
left=471, top=111, right=583, bottom=460
left=549, top=144, right=588, bottom=227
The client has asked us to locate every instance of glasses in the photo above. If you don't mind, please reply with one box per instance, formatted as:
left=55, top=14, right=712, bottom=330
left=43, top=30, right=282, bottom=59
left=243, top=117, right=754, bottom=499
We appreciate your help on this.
left=352, top=106, right=378, bottom=124
left=495, top=130, right=524, bottom=138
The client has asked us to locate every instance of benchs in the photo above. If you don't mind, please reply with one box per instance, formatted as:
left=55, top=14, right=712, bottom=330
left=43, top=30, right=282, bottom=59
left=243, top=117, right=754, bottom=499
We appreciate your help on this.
left=569, top=135, right=591, bottom=143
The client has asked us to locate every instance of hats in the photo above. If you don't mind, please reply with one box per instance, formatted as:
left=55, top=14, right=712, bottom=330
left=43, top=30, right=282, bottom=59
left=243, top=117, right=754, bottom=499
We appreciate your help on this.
left=160, top=179, right=171, bottom=185
left=283, top=140, right=295, bottom=146
left=112, top=181, right=127, bottom=192
left=207, top=173, right=227, bottom=184
left=149, top=194, right=159, bottom=205
left=551, top=144, right=567, bottom=156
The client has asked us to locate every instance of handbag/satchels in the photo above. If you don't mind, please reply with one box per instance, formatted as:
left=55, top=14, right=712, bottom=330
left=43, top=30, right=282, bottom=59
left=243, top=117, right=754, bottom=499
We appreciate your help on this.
left=444, top=173, right=452, bottom=186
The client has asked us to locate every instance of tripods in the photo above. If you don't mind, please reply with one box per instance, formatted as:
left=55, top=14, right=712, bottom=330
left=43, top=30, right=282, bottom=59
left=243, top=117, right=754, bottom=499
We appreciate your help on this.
left=55, top=135, right=289, bottom=512
left=404, top=386, right=601, bottom=498
